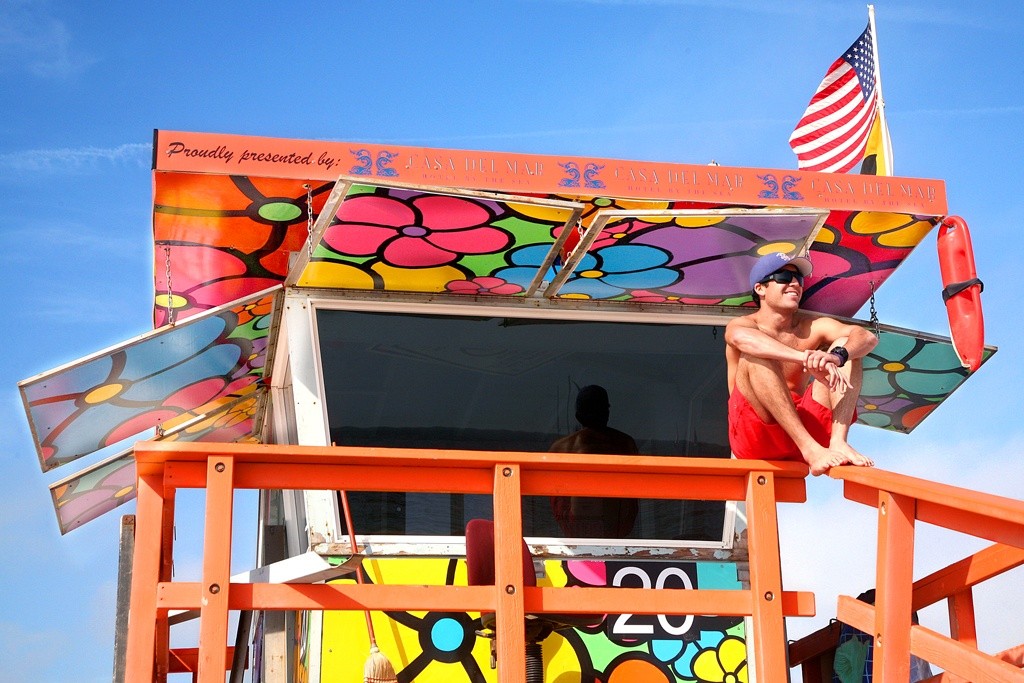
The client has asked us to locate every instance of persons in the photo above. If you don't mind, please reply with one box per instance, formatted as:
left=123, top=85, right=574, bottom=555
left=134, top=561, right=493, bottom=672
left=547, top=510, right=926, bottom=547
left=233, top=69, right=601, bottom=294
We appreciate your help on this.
left=545, top=384, right=643, bottom=539
left=833, top=588, right=933, bottom=683
left=724, top=252, right=878, bottom=477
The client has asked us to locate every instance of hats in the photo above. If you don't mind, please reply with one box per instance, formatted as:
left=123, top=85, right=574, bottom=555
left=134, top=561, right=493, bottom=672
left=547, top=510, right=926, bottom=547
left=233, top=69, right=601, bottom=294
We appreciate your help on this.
left=750, top=252, right=813, bottom=290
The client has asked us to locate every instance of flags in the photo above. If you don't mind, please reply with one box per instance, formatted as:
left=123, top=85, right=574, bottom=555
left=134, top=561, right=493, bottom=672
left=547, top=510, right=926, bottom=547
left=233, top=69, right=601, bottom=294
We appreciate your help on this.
left=788, top=19, right=878, bottom=175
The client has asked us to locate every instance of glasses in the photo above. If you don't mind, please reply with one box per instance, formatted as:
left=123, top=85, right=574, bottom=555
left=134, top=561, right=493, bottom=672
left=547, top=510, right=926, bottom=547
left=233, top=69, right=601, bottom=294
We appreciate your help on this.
left=758, top=268, right=804, bottom=287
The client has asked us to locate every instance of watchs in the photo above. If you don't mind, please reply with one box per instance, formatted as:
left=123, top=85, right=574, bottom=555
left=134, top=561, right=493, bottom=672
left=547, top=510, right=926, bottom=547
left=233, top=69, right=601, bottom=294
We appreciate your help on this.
left=830, top=346, right=849, bottom=367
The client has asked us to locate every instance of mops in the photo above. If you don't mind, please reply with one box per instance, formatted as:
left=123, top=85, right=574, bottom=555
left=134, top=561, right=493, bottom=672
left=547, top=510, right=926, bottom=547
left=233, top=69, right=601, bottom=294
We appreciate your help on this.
left=333, top=442, right=399, bottom=683
